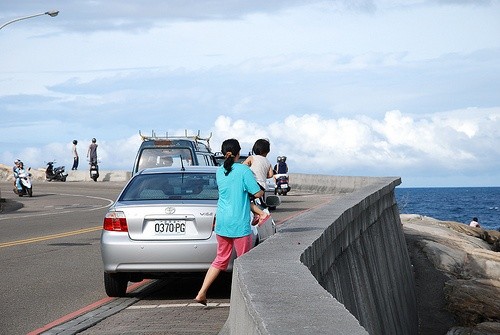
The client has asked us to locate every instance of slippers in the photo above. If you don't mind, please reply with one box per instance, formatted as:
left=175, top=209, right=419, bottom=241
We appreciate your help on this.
left=251, top=215, right=261, bottom=226
left=194, top=297, right=208, bottom=307
left=258, top=214, right=271, bottom=227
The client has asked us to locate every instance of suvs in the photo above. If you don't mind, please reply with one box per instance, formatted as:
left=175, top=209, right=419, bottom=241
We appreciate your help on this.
left=214, top=151, right=281, bottom=210
left=131, top=129, right=222, bottom=178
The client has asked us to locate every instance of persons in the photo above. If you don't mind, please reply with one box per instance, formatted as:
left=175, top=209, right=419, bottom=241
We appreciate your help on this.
left=242, top=139, right=274, bottom=228
left=194, top=138, right=263, bottom=306
left=72, top=140, right=79, bottom=170
left=470, top=218, right=480, bottom=228
left=163, top=183, right=202, bottom=194
left=12, top=159, right=31, bottom=192
left=87, top=138, right=98, bottom=164
left=273, top=156, right=289, bottom=188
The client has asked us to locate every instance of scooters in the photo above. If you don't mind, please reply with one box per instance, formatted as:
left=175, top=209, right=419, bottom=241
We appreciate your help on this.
left=273, top=169, right=288, bottom=196
left=89, top=162, right=101, bottom=182
left=13, top=167, right=33, bottom=197
left=44, top=160, right=68, bottom=182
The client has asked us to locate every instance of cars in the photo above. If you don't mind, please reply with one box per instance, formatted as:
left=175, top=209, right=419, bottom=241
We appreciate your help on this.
left=101, top=166, right=277, bottom=297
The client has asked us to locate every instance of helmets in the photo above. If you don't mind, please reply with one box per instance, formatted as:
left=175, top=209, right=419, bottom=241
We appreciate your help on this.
left=19, top=161, right=23, bottom=166
left=92, top=138, right=96, bottom=143
left=281, top=155, right=286, bottom=161
left=14, top=159, right=21, bottom=164
left=277, top=156, right=282, bottom=162
left=73, top=139, right=78, bottom=145
